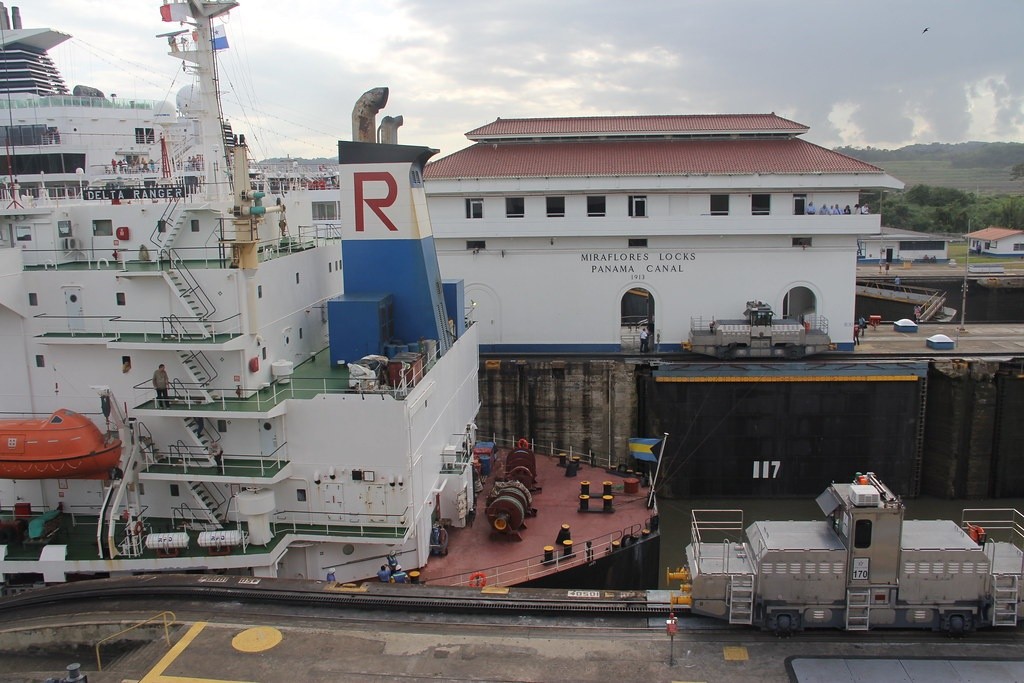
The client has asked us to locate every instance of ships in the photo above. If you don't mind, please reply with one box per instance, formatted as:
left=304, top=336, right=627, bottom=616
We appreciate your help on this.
left=0, top=0, right=670, bottom=589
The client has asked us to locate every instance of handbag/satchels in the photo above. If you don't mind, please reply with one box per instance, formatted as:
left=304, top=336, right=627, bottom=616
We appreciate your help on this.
left=862, top=324, right=867, bottom=329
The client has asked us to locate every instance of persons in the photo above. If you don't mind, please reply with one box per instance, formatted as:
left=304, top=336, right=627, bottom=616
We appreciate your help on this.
left=377, top=551, right=406, bottom=583
left=122, top=358, right=131, bottom=373
left=894, top=276, right=901, bottom=292
left=858, top=314, right=865, bottom=336
left=193, top=401, right=204, bottom=437
left=854, top=322, right=860, bottom=345
left=913, top=303, right=921, bottom=323
left=111, top=157, right=155, bottom=173
left=977, top=244, right=981, bottom=255
left=639, top=326, right=651, bottom=352
left=327, top=568, right=336, bottom=581
left=807, top=201, right=869, bottom=214
left=208, top=439, right=223, bottom=473
left=153, top=364, right=170, bottom=410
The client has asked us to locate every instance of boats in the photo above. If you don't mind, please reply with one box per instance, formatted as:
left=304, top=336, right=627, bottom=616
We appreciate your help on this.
left=665, top=471, right=1024, bottom=639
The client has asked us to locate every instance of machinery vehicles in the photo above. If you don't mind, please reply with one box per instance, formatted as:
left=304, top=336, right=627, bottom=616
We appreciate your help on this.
left=681, top=299, right=831, bottom=362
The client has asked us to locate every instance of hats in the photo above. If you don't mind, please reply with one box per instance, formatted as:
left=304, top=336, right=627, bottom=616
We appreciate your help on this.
left=328, top=567, right=335, bottom=574
left=390, top=549, right=396, bottom=556
left=395, top=564, right=402, bottom=571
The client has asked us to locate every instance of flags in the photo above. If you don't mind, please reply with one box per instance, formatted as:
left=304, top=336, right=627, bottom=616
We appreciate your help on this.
left=160, top=3, right=187, bottom=21
left=211, top=24, right=229, bottom=50
